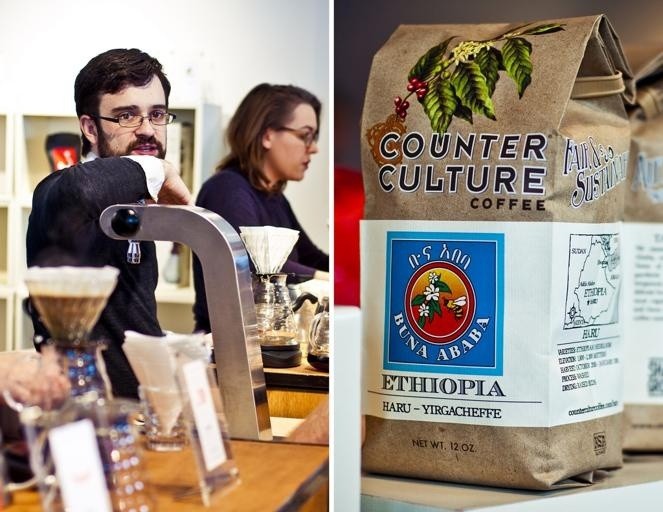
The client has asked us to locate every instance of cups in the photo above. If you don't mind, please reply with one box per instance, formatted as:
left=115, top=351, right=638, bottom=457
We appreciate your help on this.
left=138, top=385, right=188, bottom=451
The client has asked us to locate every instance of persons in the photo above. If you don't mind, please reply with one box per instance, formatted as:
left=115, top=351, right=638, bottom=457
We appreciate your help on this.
left=189, top=81, right=328, bottom=338
left=23, top=44, right=196, bottom=411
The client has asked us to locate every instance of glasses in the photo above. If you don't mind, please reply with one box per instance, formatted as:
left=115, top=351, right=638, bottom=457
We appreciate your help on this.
left=92, top=111, right=177, bottom=128
left=282, top=126, right=319, bottom=147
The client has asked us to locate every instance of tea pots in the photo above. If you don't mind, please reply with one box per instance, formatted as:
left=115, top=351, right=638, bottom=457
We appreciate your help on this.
left=250, top=273, right=301, bottom=352
